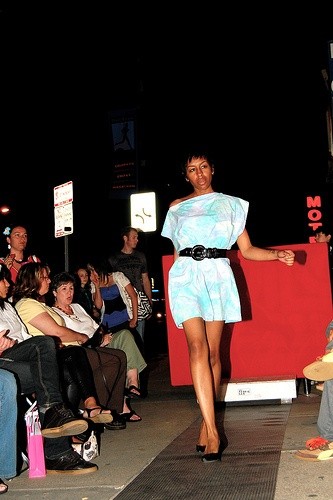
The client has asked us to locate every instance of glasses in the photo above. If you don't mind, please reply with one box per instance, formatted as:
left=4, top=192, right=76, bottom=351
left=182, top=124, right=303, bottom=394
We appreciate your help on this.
left=39, top=276, right=49, bottom=281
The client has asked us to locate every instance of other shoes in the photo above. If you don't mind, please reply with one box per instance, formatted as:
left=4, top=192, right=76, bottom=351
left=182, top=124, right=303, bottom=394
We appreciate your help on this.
left=302, top=352, right=333, bottom=381
left=45, top=451, right=98, bottom=476
left=127, top=385, right=141, bottom=399
left=196, top=443, right=205, bottom=452
left=102, top=414, right=126, bottom=430
left=201, top=439, right=222, bottom=463
left=119, top=410, right=142, bottom=422
left=39, top=403, right=88, bottom=439
left=294, top=440, right=333, bottom=462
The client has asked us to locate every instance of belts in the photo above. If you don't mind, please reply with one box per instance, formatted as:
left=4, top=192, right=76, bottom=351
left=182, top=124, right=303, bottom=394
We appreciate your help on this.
left=178, top=244, right=229, bottom=260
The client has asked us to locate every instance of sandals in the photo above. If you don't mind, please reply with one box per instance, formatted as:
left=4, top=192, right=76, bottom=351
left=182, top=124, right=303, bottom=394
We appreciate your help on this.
left=76, top=407, right=113, bottom=424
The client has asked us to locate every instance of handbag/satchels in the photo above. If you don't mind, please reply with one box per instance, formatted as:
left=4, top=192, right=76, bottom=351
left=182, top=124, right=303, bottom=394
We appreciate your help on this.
left=115, top=272, right=153, bottom=322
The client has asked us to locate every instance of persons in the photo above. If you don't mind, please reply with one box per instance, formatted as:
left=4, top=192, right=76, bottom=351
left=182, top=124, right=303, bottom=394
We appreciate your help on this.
left=314, top=225, right=333, bottom=290
left=161, top=147, right=296, bottom=464
left=295, top=320, right=333, bottom=462
left=105, top=227, right=153, bottom=400
left=0, top=223, right=151, bottom=495
